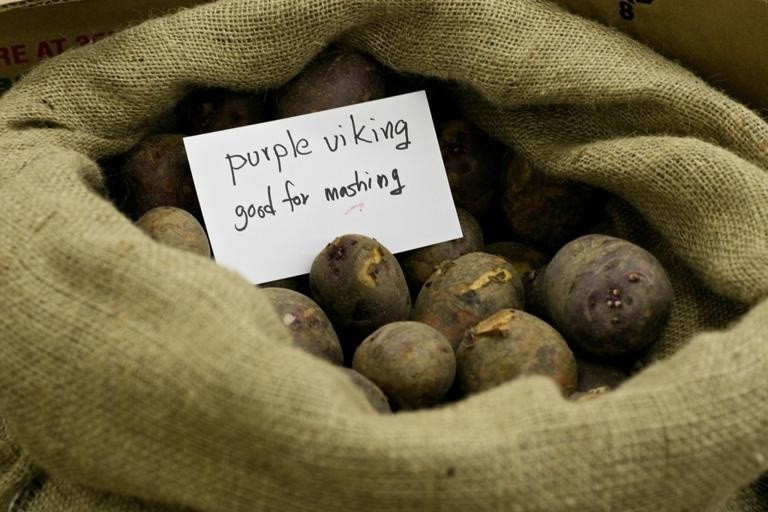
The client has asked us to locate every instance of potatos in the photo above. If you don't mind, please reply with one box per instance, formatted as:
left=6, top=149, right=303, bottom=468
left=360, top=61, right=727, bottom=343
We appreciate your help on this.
left=111, top=48, right=674, bottom=409
